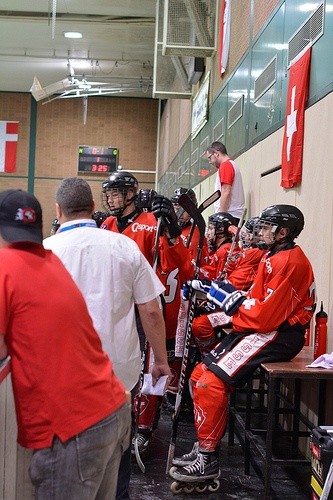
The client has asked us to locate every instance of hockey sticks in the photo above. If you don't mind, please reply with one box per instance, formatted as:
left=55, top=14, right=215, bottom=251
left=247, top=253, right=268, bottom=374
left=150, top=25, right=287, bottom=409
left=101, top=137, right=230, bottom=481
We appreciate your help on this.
left=185, top=189, right=222, bottom=248
left=219, top=208, right=248, bottom=283
left=164, top=194, right=206, bottom=479
left=133, top=216, right=163, bottom=479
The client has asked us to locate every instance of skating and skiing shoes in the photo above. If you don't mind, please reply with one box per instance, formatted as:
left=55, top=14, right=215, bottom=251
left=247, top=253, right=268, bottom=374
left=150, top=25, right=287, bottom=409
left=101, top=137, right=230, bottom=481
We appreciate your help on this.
left=168, top=451, right=222, bottom=494
left=172, top=441, right=200, bottom=467
left=131, top=432, right=152, bottom=464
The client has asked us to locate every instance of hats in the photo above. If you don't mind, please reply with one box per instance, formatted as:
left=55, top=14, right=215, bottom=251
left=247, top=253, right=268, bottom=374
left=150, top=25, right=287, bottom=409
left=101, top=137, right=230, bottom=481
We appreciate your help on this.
left=0, top=189, right=44, bottom=247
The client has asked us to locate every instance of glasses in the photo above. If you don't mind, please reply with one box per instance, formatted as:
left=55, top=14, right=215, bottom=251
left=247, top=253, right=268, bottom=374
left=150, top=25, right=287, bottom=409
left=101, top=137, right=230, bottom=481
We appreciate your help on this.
left=207, top=148, right=221, bottom=160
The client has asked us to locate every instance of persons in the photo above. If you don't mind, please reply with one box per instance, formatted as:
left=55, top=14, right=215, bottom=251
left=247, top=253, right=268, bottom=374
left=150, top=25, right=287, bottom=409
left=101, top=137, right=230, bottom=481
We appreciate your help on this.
left=0, top=189, right=132, bottom=500
left=92, top=171, right=268, bottom=452
left=169, top=204, right=317, bottom=493
left=43, top=176, right=172, bottom=500
left=207, top=142, right=245, bottom=227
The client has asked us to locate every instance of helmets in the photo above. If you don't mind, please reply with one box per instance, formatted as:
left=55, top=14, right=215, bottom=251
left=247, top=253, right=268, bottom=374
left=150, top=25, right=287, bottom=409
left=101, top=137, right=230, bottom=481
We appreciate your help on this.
left=135, top=187, right=159, bottom=213
left=257, top=204, right=305, bottom=250
left=100, top=171, right=139, bottom=217
left=171, top=186, right=198, bottom=226
left=238, top=216, right=261, bottom=248
left=207, top=211, right=238, bottom=237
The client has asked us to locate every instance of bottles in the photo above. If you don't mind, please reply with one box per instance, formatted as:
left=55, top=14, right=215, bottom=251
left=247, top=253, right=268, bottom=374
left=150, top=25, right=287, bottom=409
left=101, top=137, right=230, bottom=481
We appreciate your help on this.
left=314, top=301, right=329, bottom=359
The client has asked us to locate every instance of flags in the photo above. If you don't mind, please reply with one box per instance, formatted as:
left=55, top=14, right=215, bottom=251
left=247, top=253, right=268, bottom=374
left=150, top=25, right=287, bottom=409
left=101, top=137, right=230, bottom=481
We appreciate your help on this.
left=219, top=1, right=230, bottom=76
left=279, top=47, right=312, bottom=186
left=0, top=121, right=19, bottom=173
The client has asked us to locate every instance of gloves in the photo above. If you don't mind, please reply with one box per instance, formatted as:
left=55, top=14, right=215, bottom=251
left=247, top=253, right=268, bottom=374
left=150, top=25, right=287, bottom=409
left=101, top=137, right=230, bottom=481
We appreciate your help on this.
left=150, top=194, right=183, bottom=238
left=182, top=278, right=213, bottom=302
left=206, top=280, right=246, bottom=318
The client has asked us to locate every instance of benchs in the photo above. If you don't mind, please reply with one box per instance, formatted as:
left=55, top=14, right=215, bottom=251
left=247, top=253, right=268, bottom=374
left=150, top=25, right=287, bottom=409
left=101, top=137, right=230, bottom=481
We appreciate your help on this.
left=229, top=345, right=333, bottom=500
